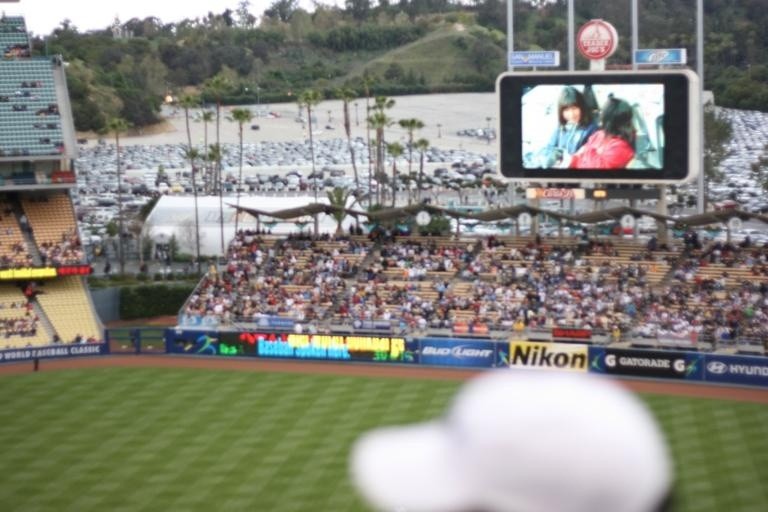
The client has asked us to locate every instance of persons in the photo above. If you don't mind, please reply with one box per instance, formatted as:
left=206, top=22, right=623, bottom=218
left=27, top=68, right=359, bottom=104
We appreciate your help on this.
left=568, top=95, right=649, bottom=173
left=0, top=25, right=99, bottom=373
left=539, top=87, right=594, bottom=164
left=184, top=224, right=767, bottom=359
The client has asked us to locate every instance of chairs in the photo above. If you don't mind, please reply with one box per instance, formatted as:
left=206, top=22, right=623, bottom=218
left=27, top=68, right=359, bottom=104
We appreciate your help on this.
left=0, top=17, right=100, bottom=350
